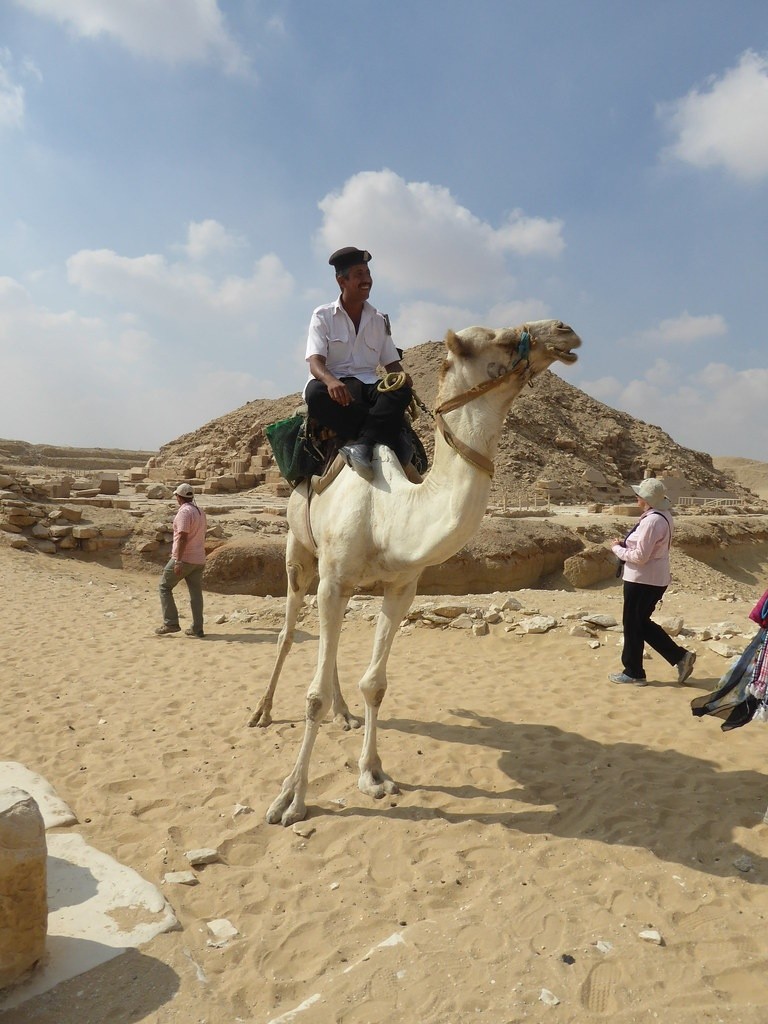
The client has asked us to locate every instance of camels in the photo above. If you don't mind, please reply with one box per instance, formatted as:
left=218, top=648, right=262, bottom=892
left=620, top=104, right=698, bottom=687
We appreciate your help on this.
left=248, top=318, right=583, bottom=827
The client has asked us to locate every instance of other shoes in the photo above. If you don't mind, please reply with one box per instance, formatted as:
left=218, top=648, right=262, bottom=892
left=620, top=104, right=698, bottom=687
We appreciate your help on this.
left=338, top=444, right=375, bottom=482
left=185, top=629, right=204, bottom=637
left=607, top=673, right=648, bottom=686
left=155, top=625, right=181, bottom=634
left=676, top=650, right=696, bottom=685
left=395, top=429, right=414, bottom=468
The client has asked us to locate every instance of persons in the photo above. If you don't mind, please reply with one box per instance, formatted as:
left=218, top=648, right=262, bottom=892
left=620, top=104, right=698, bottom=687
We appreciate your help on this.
left=304, top=247, right=428, bottom=480
left=155, top=483, right=207, bottom=637
left=608, top=478, right=696, bottom=686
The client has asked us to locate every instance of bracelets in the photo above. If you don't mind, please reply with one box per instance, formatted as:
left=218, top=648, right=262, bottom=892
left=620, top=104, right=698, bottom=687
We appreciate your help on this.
left=175, top=560, right=182, bottom=564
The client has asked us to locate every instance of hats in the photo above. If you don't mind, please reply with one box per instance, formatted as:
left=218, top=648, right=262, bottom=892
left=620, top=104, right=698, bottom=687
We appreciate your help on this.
left=328, top=247, right=383, bottom=270
left=172, top=483, right=195, bottom=497
left=631, top=478, right=671, bottom=511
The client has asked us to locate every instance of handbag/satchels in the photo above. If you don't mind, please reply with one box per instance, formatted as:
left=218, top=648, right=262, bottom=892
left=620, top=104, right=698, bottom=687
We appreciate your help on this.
left=265, top=415, right=327, bottom=482
